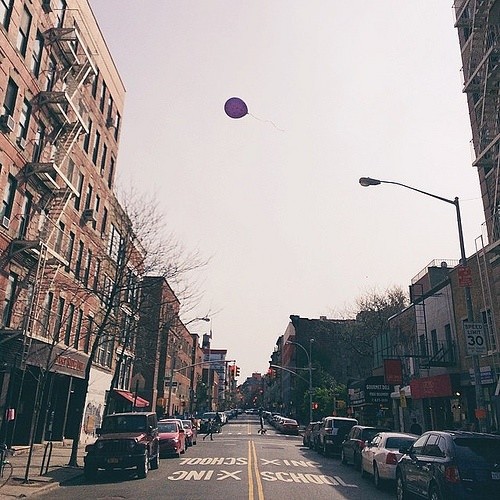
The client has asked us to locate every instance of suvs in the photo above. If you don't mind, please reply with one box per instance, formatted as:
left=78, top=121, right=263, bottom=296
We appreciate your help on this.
left=319, top=416, right=358, bottom=460
left=303, top=422, right=323, bottom=447
left=341, top=424, right=391, bottom=472
left=83, top=411, right=160, bottom=480
left=394, top=429, right=500, bottom=500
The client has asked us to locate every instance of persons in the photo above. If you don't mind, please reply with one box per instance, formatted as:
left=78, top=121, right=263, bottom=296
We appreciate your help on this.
left=203, top=418, right=213, bottom=441
left=214, top=412, right=220, bottom=422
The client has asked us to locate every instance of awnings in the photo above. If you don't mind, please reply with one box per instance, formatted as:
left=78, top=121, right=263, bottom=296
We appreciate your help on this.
left=115, top=390, right=150, bottom=407
left=410, top=373, right=471, bottom=398
left=390, top=385, right=411, bottom=398
left=349, top=375, right=394, bottom=406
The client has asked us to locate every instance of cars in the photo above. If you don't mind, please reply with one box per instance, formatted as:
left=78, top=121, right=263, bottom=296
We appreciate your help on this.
left=361, top=431, right=419, bottom=490
left=203, top=408, right=255, bottom=433
left=157, top=422, right=186, bottom=458
left=182, top=420, right=197, bottom=447
left=257, top=409, right=299, bottom=436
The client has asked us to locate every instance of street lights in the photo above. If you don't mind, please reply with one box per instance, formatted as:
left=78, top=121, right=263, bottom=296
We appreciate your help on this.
left=285, top=340, right=315, bottom=421
left=358, top=177, right=488, bottom=434
left=167, top=318, right=211, bottom=417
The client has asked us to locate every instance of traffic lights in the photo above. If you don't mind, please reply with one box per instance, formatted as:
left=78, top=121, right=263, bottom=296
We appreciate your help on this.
left=236, top=366, right=240, bottom=376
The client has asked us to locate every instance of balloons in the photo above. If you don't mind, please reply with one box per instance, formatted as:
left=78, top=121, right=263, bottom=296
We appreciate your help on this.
left=224, top=97, right=248, bottom=118
